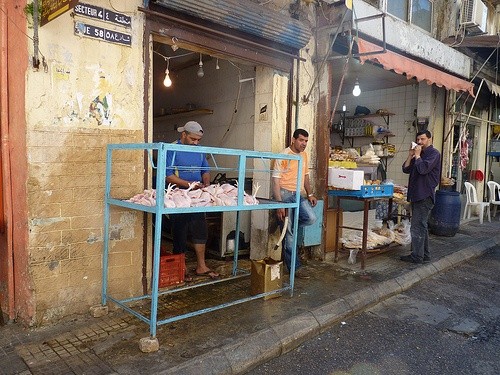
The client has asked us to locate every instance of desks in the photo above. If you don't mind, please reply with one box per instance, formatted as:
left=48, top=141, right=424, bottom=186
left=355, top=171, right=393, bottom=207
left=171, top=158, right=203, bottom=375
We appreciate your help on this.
left=334, top=196, right=414, bottom=270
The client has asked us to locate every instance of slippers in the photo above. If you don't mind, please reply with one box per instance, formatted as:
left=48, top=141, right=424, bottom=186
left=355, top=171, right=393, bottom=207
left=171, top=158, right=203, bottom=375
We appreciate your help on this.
left=195, top=270, right=220, bottom=280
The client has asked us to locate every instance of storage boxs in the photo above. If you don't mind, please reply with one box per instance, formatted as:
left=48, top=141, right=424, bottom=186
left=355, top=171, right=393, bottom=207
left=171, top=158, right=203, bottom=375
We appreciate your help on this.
left=159, top=248, right=185, bottom=287
left=327, top=167, right=393, bottom=198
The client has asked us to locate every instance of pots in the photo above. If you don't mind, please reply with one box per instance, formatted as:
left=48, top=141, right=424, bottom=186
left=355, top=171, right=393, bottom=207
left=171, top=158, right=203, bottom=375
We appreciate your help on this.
left=332, top=124, right=342, bottom=131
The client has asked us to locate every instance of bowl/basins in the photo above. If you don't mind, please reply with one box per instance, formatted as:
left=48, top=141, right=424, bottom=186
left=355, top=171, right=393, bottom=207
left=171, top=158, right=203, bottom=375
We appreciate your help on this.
left=218, top=238, right=235, bottom=253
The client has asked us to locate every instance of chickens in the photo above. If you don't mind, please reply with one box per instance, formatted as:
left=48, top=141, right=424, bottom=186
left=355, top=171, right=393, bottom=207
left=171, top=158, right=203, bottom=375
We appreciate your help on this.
left=127, top=180, right=262, bottom=209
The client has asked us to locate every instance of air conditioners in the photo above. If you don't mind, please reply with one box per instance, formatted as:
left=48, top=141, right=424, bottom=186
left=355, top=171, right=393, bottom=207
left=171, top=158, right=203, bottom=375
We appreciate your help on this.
left=459, top=0, right=488, bottom=34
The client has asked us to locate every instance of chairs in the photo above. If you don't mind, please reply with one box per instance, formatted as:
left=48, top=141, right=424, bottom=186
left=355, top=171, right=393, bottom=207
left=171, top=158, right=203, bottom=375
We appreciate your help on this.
left=485, top=181, right=500, bottom=218
left=463, top=182, right=491, bottom=224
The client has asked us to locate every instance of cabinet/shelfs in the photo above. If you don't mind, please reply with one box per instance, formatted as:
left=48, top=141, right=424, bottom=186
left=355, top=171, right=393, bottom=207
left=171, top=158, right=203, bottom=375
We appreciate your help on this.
left=105, top=143, right=303, bottom=338
left=331, top=110, right=396, bottom=183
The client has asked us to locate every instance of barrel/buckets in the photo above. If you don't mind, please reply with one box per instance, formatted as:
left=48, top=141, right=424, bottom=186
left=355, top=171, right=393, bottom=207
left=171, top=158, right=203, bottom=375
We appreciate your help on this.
left=429, top=191, right=462, bottom=236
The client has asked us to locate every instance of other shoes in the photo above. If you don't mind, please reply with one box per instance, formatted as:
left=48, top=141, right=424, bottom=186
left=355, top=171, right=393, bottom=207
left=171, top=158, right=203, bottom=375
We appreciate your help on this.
left=264, top=257, right=283, bottom=266
left=422, top=257, right=431, bottom=263
left=290, top=268, right=310, bottom=279
left=399, top=255, right=422, bottom=264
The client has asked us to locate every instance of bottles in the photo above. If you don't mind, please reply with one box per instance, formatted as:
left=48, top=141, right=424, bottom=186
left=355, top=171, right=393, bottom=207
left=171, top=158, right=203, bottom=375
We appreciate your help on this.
left=372, top=142, right=383, bottom=156
left=384, top=147, right=388, bottom=156
left=343, top=101, right=347, bottom=112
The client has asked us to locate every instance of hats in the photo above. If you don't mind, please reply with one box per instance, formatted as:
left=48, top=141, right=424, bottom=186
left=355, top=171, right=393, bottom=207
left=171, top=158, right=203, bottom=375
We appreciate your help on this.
left=177, top=121, right=204, bottom=137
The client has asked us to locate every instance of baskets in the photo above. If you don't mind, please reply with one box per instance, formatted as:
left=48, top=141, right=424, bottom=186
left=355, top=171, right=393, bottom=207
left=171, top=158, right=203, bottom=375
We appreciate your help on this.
left=159, top=253, right=185, bottom=287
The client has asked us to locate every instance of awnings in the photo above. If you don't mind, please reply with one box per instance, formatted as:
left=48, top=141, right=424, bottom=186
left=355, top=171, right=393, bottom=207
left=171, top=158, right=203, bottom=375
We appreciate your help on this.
left=340, top=31, right=475, bottom=100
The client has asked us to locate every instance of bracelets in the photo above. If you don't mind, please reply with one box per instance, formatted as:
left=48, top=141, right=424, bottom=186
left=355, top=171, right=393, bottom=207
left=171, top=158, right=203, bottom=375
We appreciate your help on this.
left=307, top=193, right=314, bottom=197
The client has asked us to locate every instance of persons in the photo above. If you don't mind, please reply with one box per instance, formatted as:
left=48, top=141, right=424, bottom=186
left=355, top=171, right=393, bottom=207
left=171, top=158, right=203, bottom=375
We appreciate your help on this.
left=272, top=129, right=317, bottom=279
left=400, top=130, right=441, bottom=263
left=164, top=121, right=220, bottom=277
